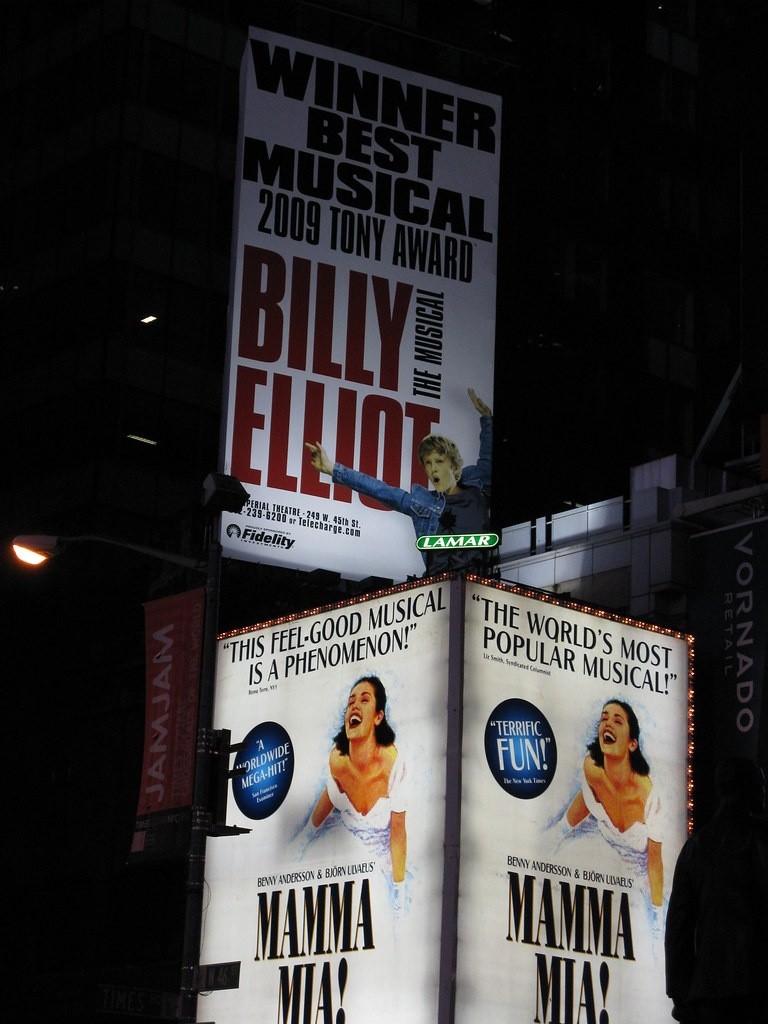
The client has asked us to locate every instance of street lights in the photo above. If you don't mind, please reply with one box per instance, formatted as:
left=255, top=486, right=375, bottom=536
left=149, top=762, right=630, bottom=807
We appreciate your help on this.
left=10, top=527, right=225, bottom=1024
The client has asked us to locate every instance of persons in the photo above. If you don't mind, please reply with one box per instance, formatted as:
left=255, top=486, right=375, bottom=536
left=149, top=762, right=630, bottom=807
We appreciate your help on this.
left=538, top=698, right=666, bottom=961
left=305, top=386, right=494, bottom=580
left=665, top=755, right=768, bottom=1024
left=289, top=676, right=408, bottom=942
